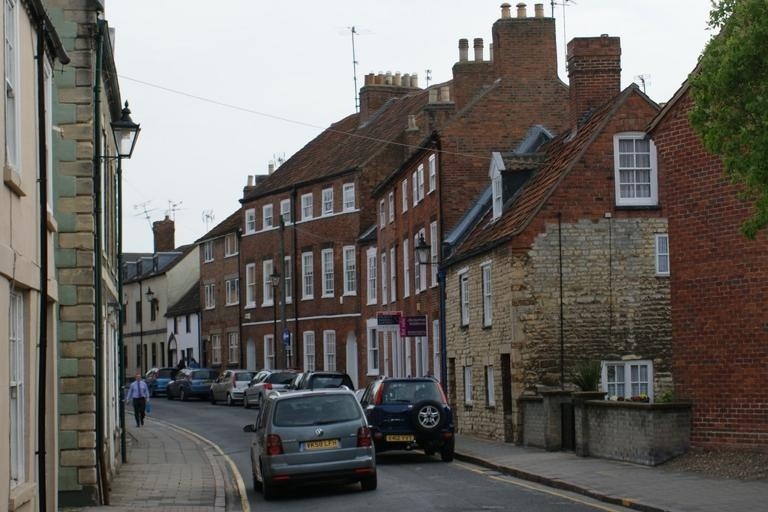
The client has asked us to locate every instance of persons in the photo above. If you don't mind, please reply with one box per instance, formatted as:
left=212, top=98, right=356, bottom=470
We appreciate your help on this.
left=126, top=372, right=151, bottom=427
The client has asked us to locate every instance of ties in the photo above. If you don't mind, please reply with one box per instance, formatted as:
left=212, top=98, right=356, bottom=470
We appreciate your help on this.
left=138, top=381, right=141, bottom=397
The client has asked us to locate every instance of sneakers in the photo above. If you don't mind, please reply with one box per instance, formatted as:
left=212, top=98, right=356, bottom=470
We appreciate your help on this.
left=136, top=421, right=144, bottom=427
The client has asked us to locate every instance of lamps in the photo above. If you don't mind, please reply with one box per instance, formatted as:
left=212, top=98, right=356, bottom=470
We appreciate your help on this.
left=414, top=233, right=443, bottom=263
left=145, top=286, right=158, bottom=303
left=100, top=101, right=140, bottom=163
